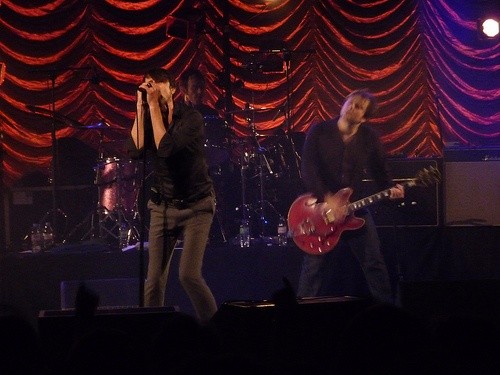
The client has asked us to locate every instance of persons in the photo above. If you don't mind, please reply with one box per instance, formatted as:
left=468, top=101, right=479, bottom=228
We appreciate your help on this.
left=297, top=89, right=404, bottom=302
left=178, top=69, right=225, bottom=180
left=131, top=69, right=217, bottom=323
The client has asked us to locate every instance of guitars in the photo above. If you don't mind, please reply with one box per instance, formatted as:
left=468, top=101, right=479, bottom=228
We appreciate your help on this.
left=288, top=161, right=444, bottom=256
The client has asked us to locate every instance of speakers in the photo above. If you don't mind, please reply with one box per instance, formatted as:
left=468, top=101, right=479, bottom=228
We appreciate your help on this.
left=61, top=277, right=142, bottom=310
left=360, top=145, right=500, bottom=228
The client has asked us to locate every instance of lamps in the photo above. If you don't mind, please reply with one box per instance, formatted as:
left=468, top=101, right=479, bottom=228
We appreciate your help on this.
left=477, top=16, right=500, bottom=40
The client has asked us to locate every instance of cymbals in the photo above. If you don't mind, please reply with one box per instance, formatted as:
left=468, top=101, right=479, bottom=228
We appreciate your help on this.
left=224, top=106, right=277, bottom=114
left=74, top=121, right=126, bottom=130
left=236, top=132, right=279, bottom=141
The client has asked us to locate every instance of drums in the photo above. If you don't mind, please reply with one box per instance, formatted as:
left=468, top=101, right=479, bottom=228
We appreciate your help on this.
left=96, top=155, right=139, bottom=216
left=239, top=145, right=287, bottom=185
left=202, top=114, right=230, bottom=165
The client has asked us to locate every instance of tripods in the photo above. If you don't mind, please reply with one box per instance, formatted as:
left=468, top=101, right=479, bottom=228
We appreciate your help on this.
left=244, top=111, right=284, bottom=234
left=24, top=67, right=141, bottom=246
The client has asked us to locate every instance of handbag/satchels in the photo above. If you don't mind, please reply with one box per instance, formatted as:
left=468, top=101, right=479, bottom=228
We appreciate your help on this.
left=171, top=174, right=197, bottom=210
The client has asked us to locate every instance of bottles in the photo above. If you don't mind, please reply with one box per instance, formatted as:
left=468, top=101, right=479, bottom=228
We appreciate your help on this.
left=240, top=218, right=250, bottom=248
left=277, top=216, right=288, bottom=246
left=31, top=223, right=42, bottom=252
left=43, top=222, right=51, bottom=251
left=118, top=223, right=128, bottom=249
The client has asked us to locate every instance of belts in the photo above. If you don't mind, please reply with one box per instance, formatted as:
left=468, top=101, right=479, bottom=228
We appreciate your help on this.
left=161, top=190, right=214, bottom=205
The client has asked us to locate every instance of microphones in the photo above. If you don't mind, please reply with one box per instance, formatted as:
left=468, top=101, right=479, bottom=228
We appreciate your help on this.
left=83, top=75, right=110, bottom=81
left=136, top=88, right=147, bottom=93
